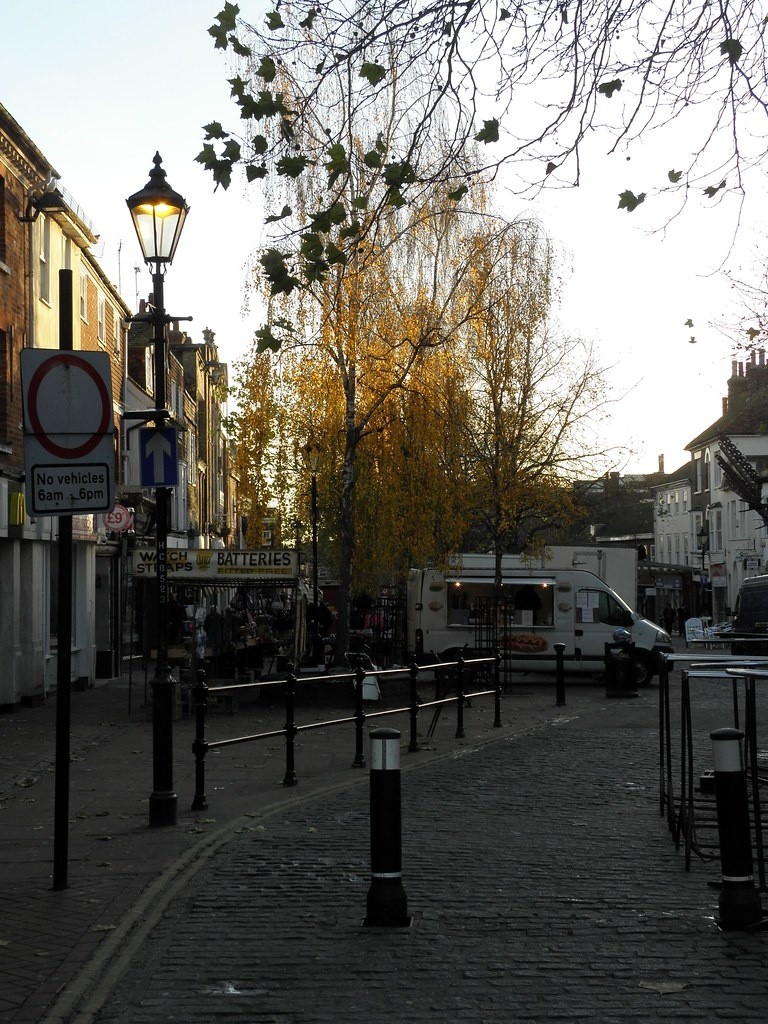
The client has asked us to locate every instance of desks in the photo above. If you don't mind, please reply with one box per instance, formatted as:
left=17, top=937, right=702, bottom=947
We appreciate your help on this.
left=151, top=635, right=285, bottom=717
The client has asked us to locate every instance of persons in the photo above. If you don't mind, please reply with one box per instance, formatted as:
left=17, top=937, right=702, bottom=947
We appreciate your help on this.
left=204, top=603, right=396, bottom=655
left=663, top=603, right=688, bottom=637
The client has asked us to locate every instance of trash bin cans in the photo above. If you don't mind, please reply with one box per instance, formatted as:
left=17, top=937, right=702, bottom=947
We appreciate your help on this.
left=604, top=642, right=639, bottom=699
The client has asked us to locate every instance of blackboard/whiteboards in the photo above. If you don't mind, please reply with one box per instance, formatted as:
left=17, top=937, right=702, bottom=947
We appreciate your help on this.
left=684, top=617, right=706, bottom=648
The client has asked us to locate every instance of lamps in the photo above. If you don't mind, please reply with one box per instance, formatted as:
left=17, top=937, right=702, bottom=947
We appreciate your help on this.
left=18, top=192, right=70, bottom=222
left=710, top=727, right=746, bottom=774
left=370, top=727, right=401, bottom=772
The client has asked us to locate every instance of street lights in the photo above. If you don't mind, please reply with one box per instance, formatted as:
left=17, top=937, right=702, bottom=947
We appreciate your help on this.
left=696, top=527, right=710, bottom=615
left=123, top=148, right=192, bottom=827
left=303, top=439, right=325, bottom=665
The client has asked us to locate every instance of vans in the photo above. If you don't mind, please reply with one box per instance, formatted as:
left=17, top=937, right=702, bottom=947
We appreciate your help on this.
left=725, top=573, right=768, bottom=655
left=406, top=566, right=676, bottom=689
